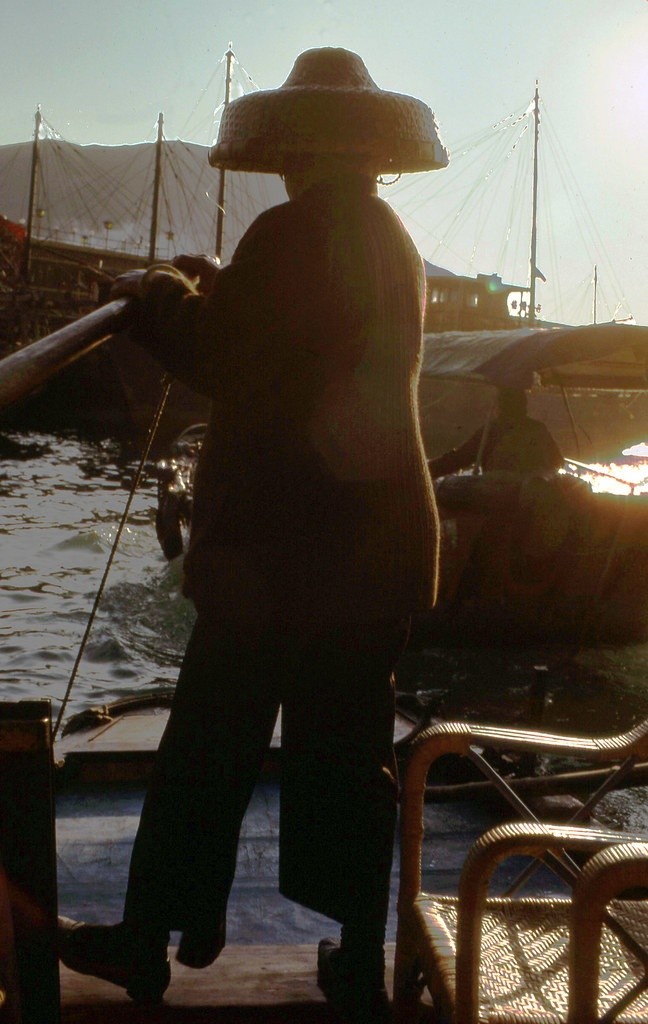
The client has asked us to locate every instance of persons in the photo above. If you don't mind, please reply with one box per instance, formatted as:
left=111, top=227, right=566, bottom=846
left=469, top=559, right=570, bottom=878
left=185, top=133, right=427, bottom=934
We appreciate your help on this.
left=58, top=50, right=441, bottom=1004
left=425, top=383, right=565, bottom=476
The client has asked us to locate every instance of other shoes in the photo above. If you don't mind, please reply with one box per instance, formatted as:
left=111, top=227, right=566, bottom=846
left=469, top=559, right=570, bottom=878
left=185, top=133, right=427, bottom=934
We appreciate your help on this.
left=55, top=922, right=172, bottom=1001
left=317, top=937, right=390, bottom=1010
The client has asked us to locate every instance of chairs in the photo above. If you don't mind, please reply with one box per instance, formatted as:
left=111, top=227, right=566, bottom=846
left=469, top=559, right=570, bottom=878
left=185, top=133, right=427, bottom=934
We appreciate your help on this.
left=393, top=718, right=648, bottom=1024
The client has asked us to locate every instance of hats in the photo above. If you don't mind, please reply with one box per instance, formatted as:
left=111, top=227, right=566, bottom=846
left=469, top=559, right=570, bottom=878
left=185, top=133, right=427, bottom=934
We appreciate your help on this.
left=208, top=47, right=449, bottom=185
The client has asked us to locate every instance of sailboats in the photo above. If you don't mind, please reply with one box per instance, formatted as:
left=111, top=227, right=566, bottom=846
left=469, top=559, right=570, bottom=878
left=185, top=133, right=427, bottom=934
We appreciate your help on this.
left=382, top=79, right=648, bottom=459
left=0, top=42, right=281, bottom=321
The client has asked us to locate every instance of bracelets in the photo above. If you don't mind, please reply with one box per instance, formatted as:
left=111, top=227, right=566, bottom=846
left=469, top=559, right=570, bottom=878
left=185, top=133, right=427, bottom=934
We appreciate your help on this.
left=142, top=263, right=183, bottom=291
